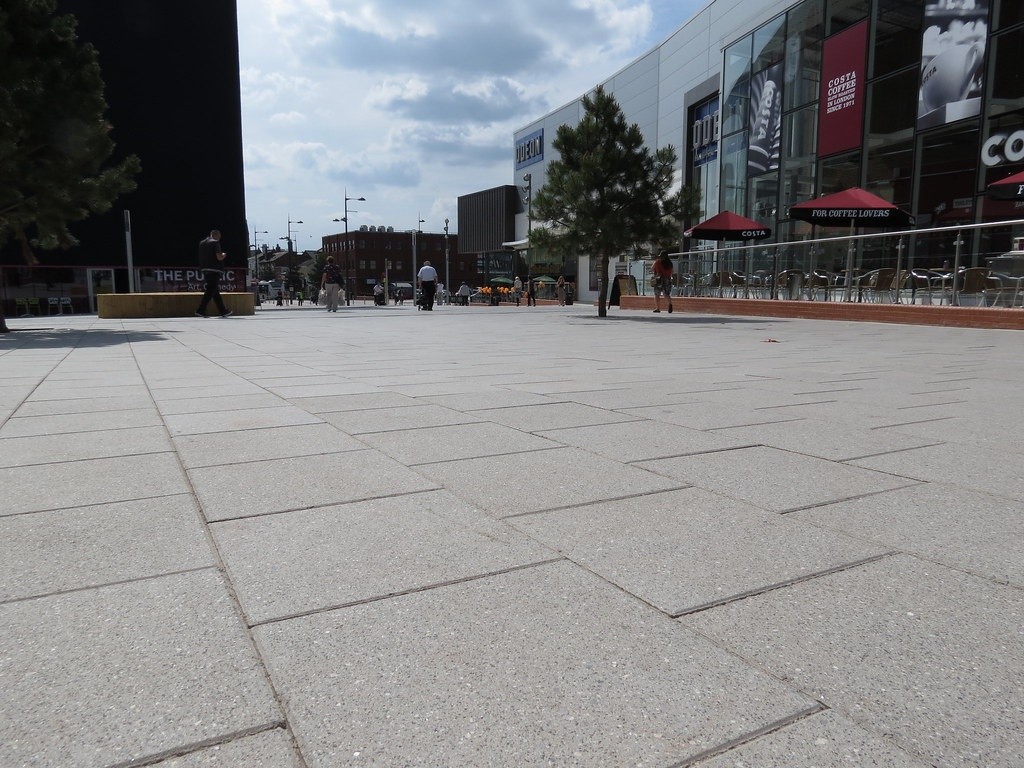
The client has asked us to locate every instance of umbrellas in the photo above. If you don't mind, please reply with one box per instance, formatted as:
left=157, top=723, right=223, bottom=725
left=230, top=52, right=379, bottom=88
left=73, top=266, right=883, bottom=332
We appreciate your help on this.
left=533, top=275, right=555, bottom=282
left=792, top=221, right=881, bottom=239
left=789, top=186, right=917, bottom=287
left=680, top=208, right=770, bottom=287
left=491, top=277, right=513, bottom=284
left=986, top=171, right=1024, bottom=203
left=936, top=193, right=1024, bottom=223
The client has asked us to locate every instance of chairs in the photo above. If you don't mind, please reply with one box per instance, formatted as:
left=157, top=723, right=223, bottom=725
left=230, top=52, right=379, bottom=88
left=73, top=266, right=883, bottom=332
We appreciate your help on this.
left=48, top=297, right=73, bottom=316
left=670, top=250, right=1024, bottom=308
left=15, top=296, right=41, bottom=316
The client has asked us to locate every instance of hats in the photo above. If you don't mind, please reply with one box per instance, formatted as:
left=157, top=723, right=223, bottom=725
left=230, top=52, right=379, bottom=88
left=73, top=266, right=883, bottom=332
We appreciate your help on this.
left=657, top=250, right=669, bottom=258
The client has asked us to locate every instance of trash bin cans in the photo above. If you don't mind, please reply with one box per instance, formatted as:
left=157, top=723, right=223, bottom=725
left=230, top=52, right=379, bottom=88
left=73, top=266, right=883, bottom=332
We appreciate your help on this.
left=565, top=292, right=574, bottom=305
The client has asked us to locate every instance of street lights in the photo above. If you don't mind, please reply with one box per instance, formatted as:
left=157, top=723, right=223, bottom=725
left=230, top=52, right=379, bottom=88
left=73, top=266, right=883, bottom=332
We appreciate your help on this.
left=249, top=227, right=269, bottom=279
left=443, top=218, right=451, bottom=305
left=332, top=187, right=366, bottom=307
left=278, top=212, right=303, bottom=305
left=409, top=212, right=426, bottom=268
left=521, top=174, right=532, bottom=275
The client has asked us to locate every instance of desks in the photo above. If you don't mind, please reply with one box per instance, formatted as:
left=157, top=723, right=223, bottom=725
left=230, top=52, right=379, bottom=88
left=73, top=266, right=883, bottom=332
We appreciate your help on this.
left=984, top=257, right=1024, bottom=307
left=927, top=268, right=957, bottom=305
left=840, top=267, right=872, bottom=301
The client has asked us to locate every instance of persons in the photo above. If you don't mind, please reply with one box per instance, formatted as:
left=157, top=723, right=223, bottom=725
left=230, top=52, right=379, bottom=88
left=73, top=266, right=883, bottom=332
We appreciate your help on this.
left=322, top=255, right=341, bottom=312
left=372, top=282, right=386, bottom=306
left=649, top=249, right=673, bottom=313
left=526, top=275, right=536, bottom=307
left=416, top=260, right=438, bottom=312
left=556, top=276, right=566, bottom=306
left=513, top=276, right=523, bottom=295
left=436, top=281, right=444, bottom=305
left=277, top=289, right=282, bottom=298
left=459, top=281, right=471, bottom=305
left=394, top=288, right=405, bottom=306
left=313, top=288, right=319, bottom=306
left=296, top=290, right=304, bottom=306
left=194, top=229, right=234, bottom=318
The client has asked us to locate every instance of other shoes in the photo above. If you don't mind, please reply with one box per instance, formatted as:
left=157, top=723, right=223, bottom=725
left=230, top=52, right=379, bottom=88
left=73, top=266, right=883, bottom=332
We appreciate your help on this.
left=195, top=310, right=208, bottom=317
left=653, top=309, right=661, bottom=313
left=668, top=303, right=673, bottom=313
left=222, top=310, right=235, bottom=317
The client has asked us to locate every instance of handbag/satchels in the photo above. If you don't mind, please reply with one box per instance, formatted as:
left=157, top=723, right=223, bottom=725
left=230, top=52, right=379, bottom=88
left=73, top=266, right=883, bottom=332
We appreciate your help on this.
left=337, top=289, right=348, bottom=307
left=651, top=275, right=663, bottom=287
left=319, top=288, right=328, bottom=305
left=337, top=274, right=345, bottom=288
